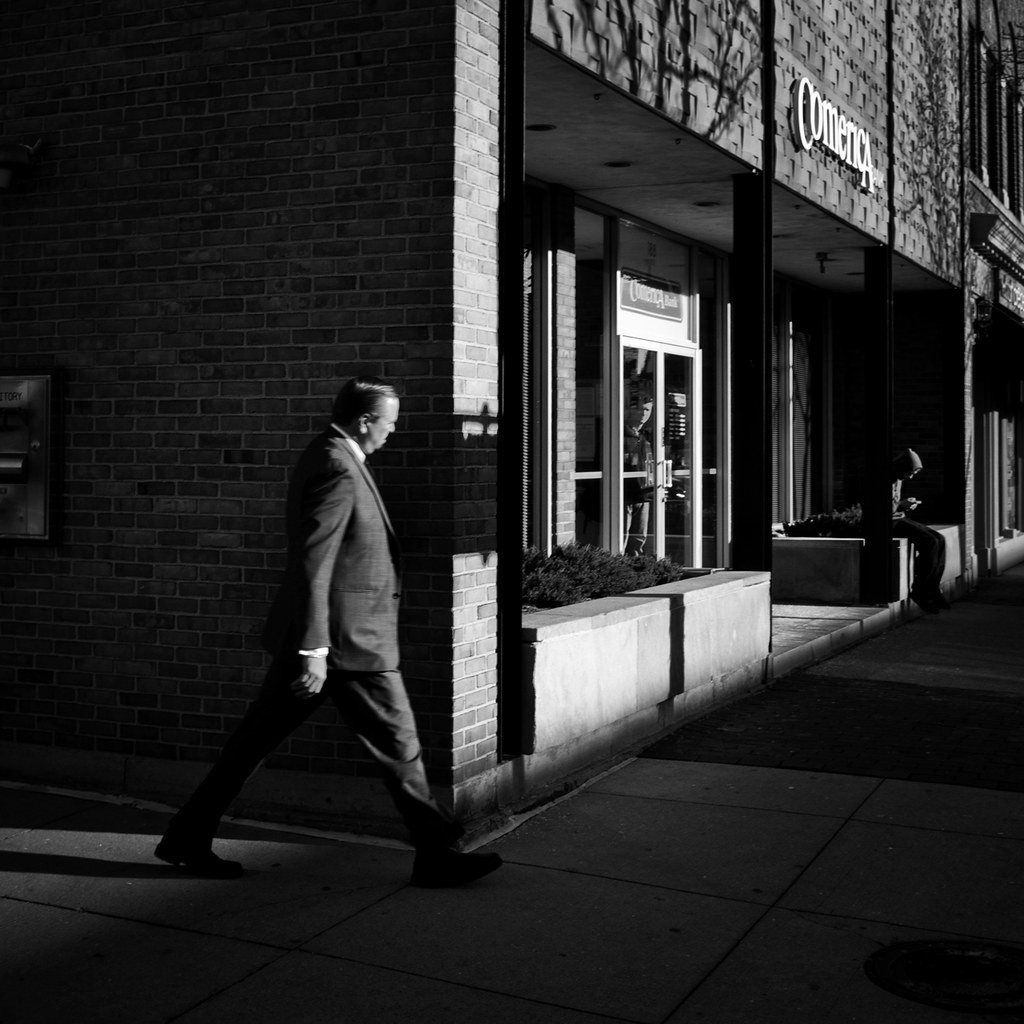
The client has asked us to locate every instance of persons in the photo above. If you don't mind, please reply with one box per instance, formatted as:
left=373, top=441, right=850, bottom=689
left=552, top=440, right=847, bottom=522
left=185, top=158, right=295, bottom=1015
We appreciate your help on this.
left=622, top=391, right=656, bottom=557
left=860, top=446, right=951, bottom=621
left=154, top=379, right=503, bottom=887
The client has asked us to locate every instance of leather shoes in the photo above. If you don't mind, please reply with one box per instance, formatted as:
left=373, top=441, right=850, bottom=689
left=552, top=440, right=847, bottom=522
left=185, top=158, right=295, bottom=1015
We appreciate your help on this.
left=155, top=832, right=245, bottom=879
left=411, top=847, right=504, bottom=888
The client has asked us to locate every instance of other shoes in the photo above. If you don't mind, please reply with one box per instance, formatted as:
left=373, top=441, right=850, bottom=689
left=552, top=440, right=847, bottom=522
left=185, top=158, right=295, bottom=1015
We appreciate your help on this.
left=912, top=585, right=951, bottom=615
